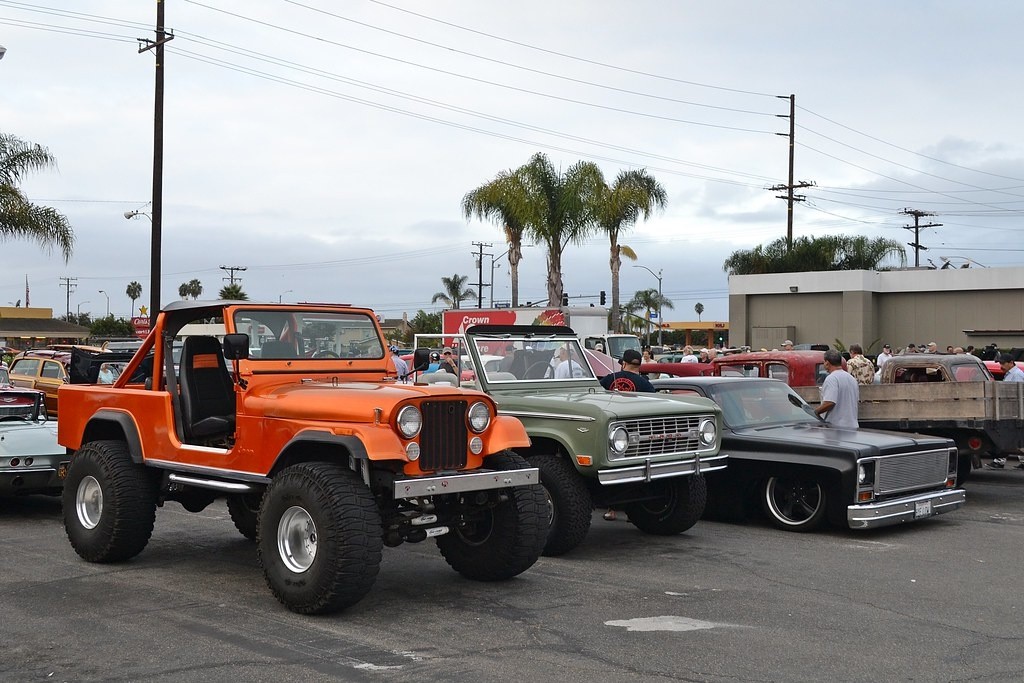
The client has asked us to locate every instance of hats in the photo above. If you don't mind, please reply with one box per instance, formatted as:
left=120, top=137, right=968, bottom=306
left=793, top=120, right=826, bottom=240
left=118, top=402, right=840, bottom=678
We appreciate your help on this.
left=908, top=343, right=915, bottom=348
left=430, top=352, right=440, bottom=360
left=595, top=343, right=603, bottom=352
left=618, top=350, right=641, bottom=365
left=995, top=353, right=1013, bottom=363
left=918, top=344, right=926, bottom=348
left=928, top=342, right=936, bottom=346
left=781, top=340, right=793, bottom=346
left=700, top=347, right=708, bottom=354
left=443, top=347, right=452, bottom=354
left=883, top=344, right=891, bottom=349
left=506, top=345, right=517, bottom=351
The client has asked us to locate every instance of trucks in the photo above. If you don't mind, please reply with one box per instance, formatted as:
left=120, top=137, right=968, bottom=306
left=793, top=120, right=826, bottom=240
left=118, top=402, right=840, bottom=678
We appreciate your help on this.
left=579, top=334, right=650, bottom=360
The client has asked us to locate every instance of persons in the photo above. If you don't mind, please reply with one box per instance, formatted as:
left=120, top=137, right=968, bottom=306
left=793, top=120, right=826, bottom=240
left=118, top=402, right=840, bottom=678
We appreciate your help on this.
left=98, top=363, right=114, bottom=384
left=499, top=344, right=533, bottom=373
left=718, top=340, right=793, bottom=372
left=599, top=349, right=656, bottom=521
left=847, top=344, right=875, bottom=385
left=984, top=354, right=1024, bottom=469
left=423, top=347, right=462, bottom=385
left=814, top=349, right=859, bottom=429
left=0, top=358, right=8, bottom=369
left=876, top=341, right=975, bottom=379
left=989, top=343, right=1001, bottom=361
left=681, top=345, right=717, bottom=364
left=641, top=345, right=660, bottom=380
left=347, top=352, right=361, bottom=358
left=368, top=346, right=382, bottom=359
left=388, top=345, right=410, bottom=381
left=594, top=344, right=603, bottom=353
left=544, top=343, right=588, bottom=379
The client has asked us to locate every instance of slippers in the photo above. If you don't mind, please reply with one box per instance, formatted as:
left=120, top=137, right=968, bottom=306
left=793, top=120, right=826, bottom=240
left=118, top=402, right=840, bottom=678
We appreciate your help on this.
left=603, top=511, right=617, bottom=521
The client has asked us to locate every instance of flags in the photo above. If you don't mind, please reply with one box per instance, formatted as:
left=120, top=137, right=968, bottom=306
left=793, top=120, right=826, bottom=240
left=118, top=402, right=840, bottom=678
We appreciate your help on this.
left=25, top=275, right=31, bottom=305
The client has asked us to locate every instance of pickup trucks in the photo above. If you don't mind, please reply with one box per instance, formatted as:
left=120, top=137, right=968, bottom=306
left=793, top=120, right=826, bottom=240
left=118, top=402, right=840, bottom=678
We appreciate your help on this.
left=57, top=300, right=553, bottom=617
left=0, top=361, right=47, bottom=423
left=637, top=363, right=1024, bottom=490
left=410, top=322, right=729, bottom=556
left=649, top=376, right=967, bottom=532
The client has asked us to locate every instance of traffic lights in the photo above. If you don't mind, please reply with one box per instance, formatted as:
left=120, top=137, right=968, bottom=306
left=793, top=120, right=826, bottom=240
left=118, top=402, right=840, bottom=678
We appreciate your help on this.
left=563, top=293, right=568, bottom=306
left=600, top=291, right=607, bottom=305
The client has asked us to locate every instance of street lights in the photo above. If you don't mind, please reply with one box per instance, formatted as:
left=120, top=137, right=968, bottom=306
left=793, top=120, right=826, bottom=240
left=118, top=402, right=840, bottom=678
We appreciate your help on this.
left=124, top=211, right=161, bottom=389
left=633, top=265, right=661, bottom=345
left=491, top=244, right=534, bottom=308
left=78, top=302, right=90, bottom=317
left=98, top=291, right=110, bottom=317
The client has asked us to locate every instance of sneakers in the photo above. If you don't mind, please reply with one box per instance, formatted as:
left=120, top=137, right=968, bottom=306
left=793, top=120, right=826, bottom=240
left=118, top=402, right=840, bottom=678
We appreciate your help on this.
left=1012, top=463, right=1024, bottom=469
left=985, top=462, right=1004, bottom=469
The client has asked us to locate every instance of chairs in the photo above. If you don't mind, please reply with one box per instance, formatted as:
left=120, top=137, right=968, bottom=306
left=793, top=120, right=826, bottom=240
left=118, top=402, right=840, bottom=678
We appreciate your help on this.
left=178, top=336, right=237, bottom=436
left=261, top=340, right=296, bottom=358
left=416, top=371, right=458, bottom=388
left=475, top=372, right=517, bottom=392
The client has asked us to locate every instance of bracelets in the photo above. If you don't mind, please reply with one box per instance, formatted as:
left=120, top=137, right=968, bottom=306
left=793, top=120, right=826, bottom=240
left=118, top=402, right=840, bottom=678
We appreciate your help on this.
left=452, top=365, right=456, bottom=369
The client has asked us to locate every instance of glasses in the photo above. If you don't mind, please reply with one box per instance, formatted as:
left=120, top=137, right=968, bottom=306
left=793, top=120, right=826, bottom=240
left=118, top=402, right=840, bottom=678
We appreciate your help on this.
left=443, top=352, right=451, bottom=356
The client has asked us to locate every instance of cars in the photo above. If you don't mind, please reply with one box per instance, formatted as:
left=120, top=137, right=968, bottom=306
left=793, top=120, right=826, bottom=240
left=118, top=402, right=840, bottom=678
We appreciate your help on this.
left=710, top=352, right=847, bottom=388
left=9, top=350, right=121, bottom=417
left=881, top=355, right=1024, bottom=382
left=641, top=345, right=743, bottom=363
left=0, top=390, right=72, bottom=516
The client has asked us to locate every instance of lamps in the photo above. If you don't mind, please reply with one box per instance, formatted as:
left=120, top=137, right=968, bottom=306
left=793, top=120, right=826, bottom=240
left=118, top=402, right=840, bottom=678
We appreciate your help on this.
left=790, top=287, right=798, bottom=291
